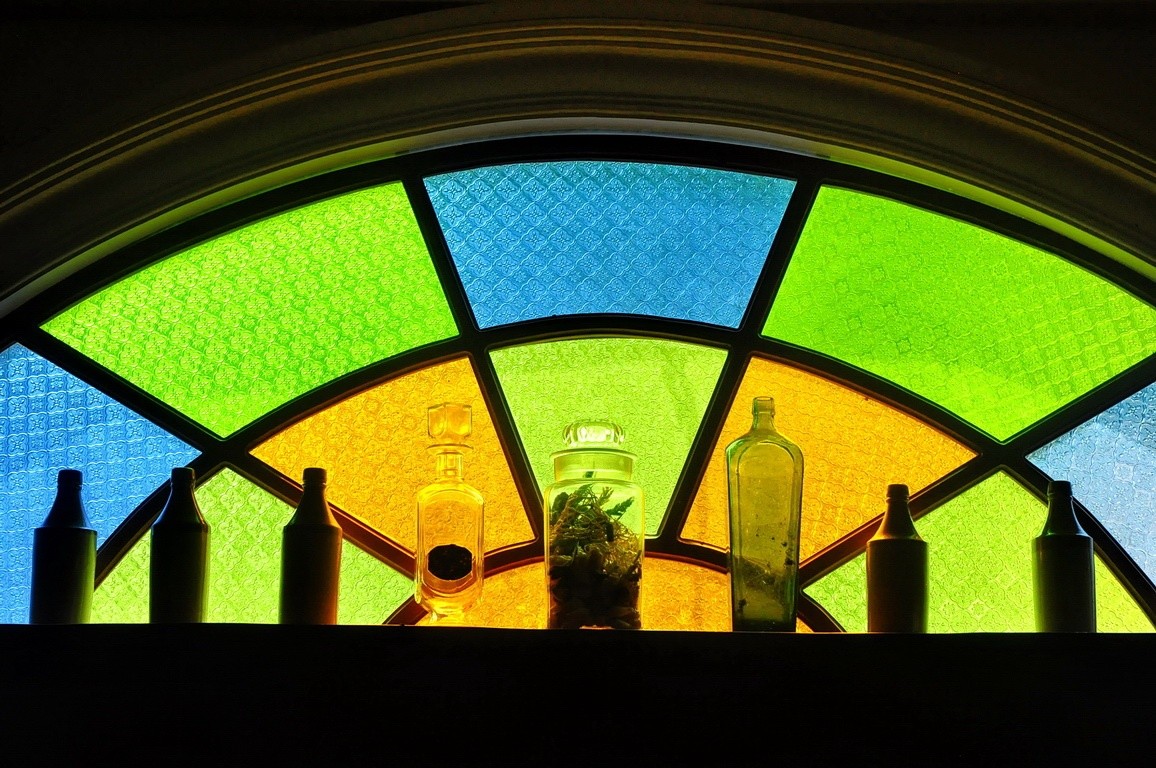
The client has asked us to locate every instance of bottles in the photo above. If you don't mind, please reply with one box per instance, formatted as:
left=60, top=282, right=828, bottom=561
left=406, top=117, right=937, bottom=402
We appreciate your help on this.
left=29, top=470, right=97, bottom=624
left=279, top=467, right=341, bottom=624
left=1031, top=479, right=1098, bottom=633
left=724, top=395, right=804, bottom=632
left=542, top=418, right=645, bottom=632
left=149, top=466, right=212, bottom=622
left=865, top=482, right=929, bottom=633
left=412, top=403, right=484, bottom=625
